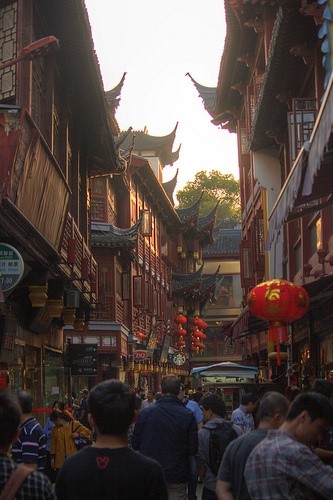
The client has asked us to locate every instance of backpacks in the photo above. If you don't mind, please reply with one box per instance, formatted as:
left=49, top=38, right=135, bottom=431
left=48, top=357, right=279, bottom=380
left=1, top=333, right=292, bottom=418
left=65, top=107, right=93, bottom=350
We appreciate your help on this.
left=202, top=422, right=238, bottom=476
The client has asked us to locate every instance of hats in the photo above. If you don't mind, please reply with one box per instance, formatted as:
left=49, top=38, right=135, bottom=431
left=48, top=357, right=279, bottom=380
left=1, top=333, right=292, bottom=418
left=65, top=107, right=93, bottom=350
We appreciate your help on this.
left=80, top=389, right=88, bottom=394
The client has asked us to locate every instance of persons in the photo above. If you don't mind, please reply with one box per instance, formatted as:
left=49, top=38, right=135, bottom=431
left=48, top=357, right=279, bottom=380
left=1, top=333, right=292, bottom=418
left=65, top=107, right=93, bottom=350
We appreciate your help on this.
left=0, top=389, right=95, bottom=500
left=130, top=375, right=211, bottom=500
left=55, top=379, right=169, bottom=500
left=198, top=394, right=244, bottom=500
left=215, top=379, right=333, bottom=500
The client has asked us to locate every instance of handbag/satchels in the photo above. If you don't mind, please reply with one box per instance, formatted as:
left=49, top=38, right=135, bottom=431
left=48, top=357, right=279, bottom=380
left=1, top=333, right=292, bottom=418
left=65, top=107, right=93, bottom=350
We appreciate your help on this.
left=72, top=420, right=92, bottom=451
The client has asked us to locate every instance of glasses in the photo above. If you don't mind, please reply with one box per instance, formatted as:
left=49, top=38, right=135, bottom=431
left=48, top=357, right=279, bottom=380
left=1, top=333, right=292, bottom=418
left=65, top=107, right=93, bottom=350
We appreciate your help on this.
left=200, top=407, right=207, bottom=411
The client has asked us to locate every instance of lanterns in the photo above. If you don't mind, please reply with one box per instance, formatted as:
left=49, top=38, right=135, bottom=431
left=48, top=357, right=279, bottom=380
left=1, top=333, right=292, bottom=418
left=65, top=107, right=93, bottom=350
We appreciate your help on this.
left=174, top=316, right=187, bottom=349
left=248, top=279, right=309, bottom=365
left=189, top=318, right=207, bottom=352
